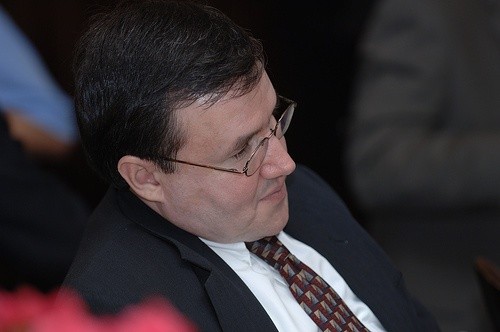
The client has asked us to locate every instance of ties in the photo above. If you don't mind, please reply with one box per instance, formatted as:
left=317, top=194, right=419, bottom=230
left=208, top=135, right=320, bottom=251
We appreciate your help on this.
left=244, top=235, right=372, bottom=332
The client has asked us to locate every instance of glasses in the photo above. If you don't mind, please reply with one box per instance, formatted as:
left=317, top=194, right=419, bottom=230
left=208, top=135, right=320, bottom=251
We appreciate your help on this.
left=144, top=96, right=297, bottom=177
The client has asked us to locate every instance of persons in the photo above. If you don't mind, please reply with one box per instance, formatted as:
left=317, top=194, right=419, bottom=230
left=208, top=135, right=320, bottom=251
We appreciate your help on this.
left=347, top=0, right=500, bottom=332
left=0, top=0, right=91, bottom=297
left=48, top=0, right=451, bottom=332
